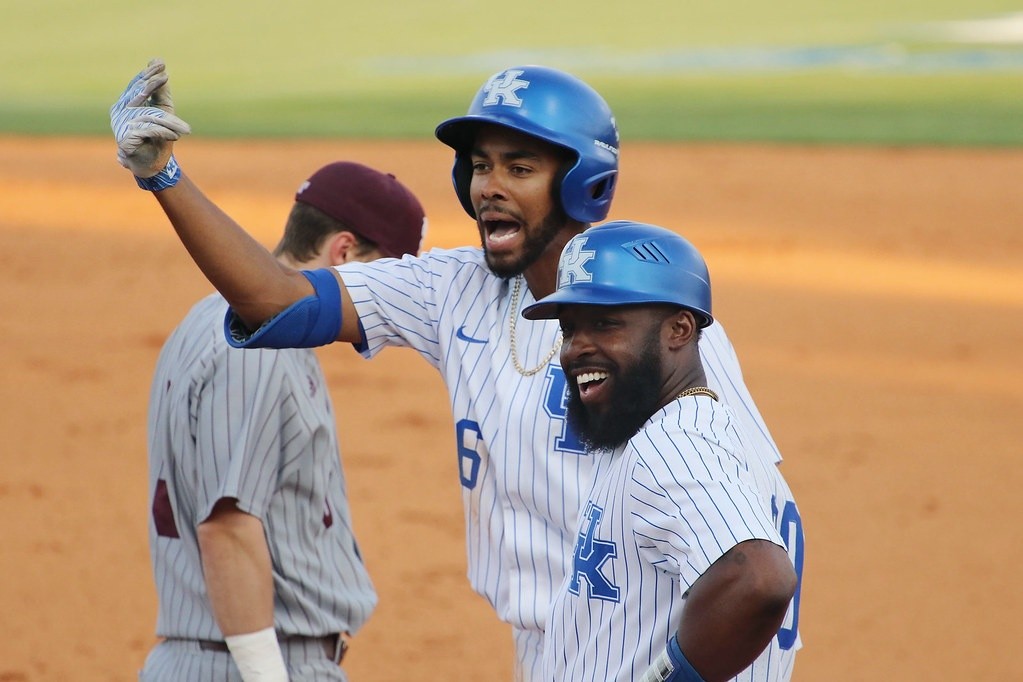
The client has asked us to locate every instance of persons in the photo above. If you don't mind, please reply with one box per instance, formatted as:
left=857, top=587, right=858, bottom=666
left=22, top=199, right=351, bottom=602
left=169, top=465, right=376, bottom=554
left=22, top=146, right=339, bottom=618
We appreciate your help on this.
left=109, top=57, right=783, bottom=682
left=140, top=162, right=427, bottom=682
left=521, top=222, right=805, bottom=682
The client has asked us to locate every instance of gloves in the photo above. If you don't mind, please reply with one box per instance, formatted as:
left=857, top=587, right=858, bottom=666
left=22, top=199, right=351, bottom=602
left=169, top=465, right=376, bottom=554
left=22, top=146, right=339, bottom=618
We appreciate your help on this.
left=109, top=58, right=191, bottom=190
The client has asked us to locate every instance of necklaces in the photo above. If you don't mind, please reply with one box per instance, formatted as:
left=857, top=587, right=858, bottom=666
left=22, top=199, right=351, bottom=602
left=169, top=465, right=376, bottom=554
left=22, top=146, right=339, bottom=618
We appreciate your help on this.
left=510, top=274, right=563, bottom=376
left=676, top=387, right=719, bottom=402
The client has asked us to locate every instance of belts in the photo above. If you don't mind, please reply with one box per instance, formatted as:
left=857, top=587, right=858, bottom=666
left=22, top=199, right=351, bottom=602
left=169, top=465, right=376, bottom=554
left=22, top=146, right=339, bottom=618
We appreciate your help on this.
left=198, top=633, right=348, bottom=665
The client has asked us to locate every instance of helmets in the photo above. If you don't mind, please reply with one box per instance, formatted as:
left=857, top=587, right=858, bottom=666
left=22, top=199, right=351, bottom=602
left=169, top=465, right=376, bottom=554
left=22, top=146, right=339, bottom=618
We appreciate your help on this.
left=434, top=64, right=619, bottom=223
left=522, top=220, right=715, bottom=329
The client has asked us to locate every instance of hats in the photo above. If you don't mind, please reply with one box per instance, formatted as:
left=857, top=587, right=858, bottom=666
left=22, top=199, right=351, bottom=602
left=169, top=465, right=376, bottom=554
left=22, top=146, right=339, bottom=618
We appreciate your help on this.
left=296, top=158, right=426, bottom=259
